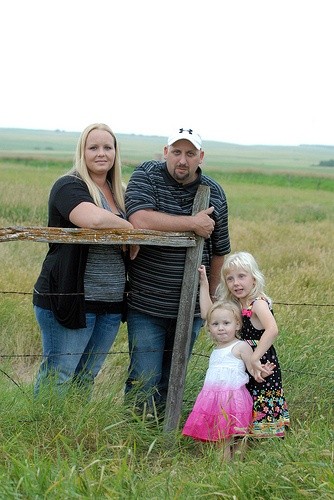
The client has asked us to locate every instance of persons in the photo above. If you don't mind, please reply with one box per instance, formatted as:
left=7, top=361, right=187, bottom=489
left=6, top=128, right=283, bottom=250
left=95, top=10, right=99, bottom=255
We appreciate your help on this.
left=32, top=124, right=138, bottom=459
left=181, top=301, right=277, bottom=459
left=122, top=126, right=231, bottom=454
left=198, top=252, right=291, bottom=459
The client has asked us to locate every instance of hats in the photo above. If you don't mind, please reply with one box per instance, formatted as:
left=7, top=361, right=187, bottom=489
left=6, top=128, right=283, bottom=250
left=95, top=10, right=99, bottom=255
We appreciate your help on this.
left=168, top=127, right=202, bottom=151
